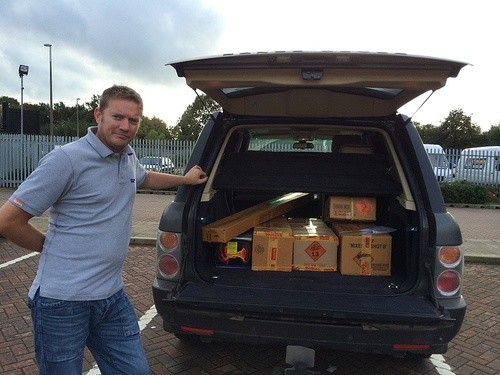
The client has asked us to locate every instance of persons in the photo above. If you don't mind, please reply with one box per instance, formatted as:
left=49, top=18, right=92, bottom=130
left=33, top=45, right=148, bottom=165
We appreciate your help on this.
left=0, top=86, right=209, bottom=375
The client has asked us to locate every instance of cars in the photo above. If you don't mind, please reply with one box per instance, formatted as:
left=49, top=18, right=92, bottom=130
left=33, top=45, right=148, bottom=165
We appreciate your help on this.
left=151, top=50, right=475, bottom=363
left=139, top=156, right=176, bottom=174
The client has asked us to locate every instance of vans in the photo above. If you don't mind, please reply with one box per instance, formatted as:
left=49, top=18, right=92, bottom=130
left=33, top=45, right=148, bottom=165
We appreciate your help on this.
left=455, top=146, right=500, bottom=185
left=423, top=144, right=452, bottom=182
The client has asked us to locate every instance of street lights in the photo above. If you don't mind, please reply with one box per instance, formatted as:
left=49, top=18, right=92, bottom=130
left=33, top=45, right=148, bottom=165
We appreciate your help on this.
left=76, top=97, right=82, bottom=137
left=19, top=64, right=29, bottom=134
left=43, top=43, right=55, bottom=152
